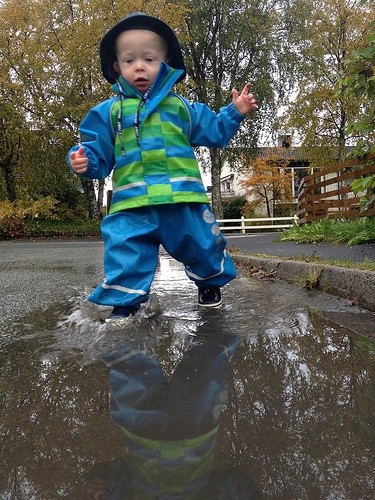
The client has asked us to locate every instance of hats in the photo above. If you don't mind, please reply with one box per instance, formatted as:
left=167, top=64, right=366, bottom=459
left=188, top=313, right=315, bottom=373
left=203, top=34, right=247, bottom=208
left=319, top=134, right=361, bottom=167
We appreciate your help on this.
left=100, top=11, right=186, bottom=85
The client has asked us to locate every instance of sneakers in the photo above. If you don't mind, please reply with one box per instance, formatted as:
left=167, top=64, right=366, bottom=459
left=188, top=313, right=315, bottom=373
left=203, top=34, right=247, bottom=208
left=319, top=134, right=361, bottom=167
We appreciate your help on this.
left=198, top=286, right=222, bottom=307
left=105, top=304, right=141, bottom=322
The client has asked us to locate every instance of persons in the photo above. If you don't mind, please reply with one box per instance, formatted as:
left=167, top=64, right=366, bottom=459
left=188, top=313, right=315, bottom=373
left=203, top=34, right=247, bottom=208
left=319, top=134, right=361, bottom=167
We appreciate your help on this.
left=89, top=322, right=259, bottom=500
left=67, top=13, right=258, bottom=322
